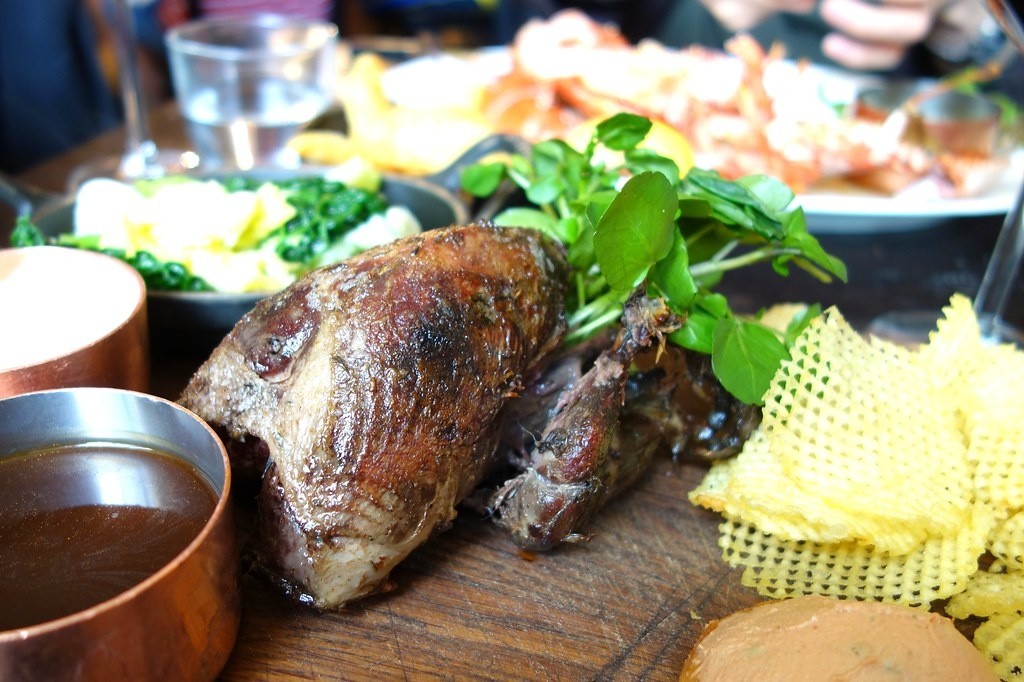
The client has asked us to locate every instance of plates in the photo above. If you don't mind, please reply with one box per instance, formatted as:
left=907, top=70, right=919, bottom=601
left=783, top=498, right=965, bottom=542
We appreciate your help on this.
left=395, top=41, right=1021, bottom=232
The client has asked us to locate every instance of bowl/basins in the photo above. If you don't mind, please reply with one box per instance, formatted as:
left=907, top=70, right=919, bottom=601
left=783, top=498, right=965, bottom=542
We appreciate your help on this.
left=0, top=132, right=537, bottom=333
left=1, top=385, right=243, bottom=682
left=0, top=246, right=151, bottom=397
left=856, top=81, right=1003, bottom=159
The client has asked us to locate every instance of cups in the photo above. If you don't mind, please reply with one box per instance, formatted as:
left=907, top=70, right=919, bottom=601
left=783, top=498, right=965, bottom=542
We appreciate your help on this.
left=163, top=10, right=341, bottom=166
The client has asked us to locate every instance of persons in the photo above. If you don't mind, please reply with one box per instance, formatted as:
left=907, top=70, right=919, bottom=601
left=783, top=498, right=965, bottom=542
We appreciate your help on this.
left=658, top=1, right=1024, bottom=98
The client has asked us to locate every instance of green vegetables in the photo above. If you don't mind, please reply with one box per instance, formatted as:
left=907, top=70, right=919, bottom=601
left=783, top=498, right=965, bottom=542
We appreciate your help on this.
left=459, top=113, right=853, bottom=426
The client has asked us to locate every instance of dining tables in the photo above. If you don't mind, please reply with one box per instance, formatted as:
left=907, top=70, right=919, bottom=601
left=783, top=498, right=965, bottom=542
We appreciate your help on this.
left=0, top=36, right=1024, bottom=680
